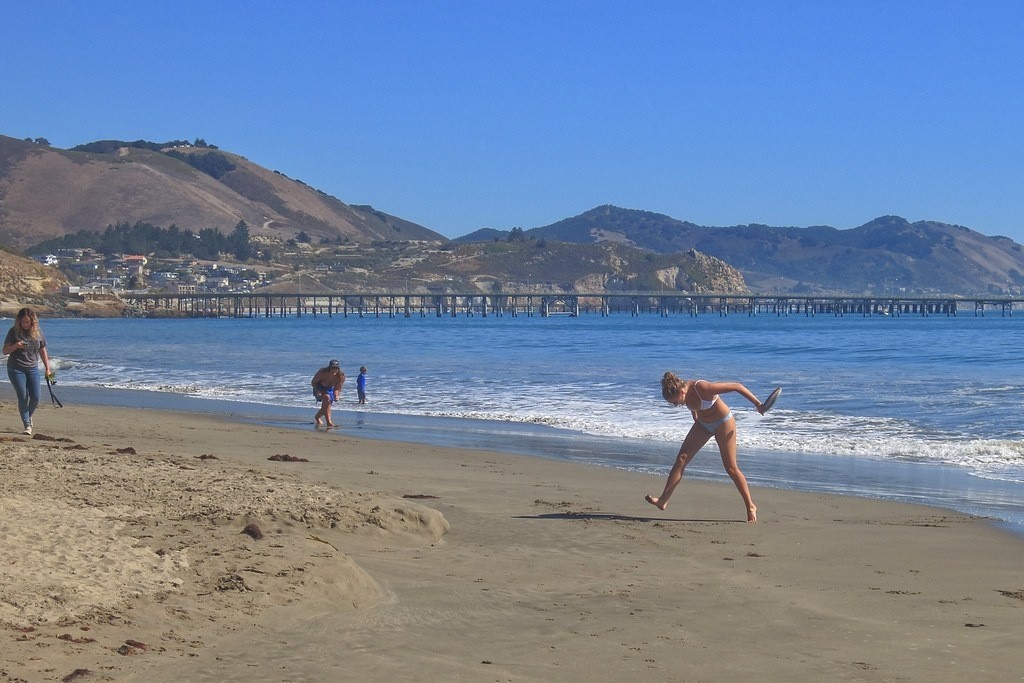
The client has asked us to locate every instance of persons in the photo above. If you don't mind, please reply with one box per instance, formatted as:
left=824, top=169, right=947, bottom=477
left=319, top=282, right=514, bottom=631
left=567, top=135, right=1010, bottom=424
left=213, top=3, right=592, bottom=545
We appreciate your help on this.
left=312, top=360, right=345, bottom=426
left=3, top=307, right=52, bottom=434
left=645, top=372, right=765, bottom=523
left=357, top=366, right=367, bottom=404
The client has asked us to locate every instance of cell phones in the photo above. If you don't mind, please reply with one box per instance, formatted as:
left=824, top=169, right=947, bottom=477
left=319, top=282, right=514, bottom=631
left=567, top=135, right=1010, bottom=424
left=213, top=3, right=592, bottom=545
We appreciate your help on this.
left=22, top=343, right=28, bottom=346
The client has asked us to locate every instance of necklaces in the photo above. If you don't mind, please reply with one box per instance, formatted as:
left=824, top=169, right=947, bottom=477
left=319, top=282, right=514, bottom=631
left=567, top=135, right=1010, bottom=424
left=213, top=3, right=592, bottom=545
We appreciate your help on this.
left=682, top=389, right=690, bottom=405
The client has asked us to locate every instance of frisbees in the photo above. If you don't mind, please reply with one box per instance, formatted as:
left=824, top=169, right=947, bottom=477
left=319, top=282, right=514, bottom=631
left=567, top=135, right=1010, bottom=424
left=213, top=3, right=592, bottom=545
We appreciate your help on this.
left=762, top=387, right=783, bottom=414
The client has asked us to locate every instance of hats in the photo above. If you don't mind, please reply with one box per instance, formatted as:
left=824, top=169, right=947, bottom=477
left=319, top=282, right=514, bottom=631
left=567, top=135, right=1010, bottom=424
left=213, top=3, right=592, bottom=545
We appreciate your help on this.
left=329, top=360, right=339, bottom=368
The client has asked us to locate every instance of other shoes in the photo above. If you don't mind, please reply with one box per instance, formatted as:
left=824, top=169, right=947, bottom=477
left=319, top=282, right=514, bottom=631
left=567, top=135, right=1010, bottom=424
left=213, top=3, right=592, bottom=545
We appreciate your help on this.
left=29, top=418, right=33, bottom=428
left=23, top=426, right=32, bottom=435
